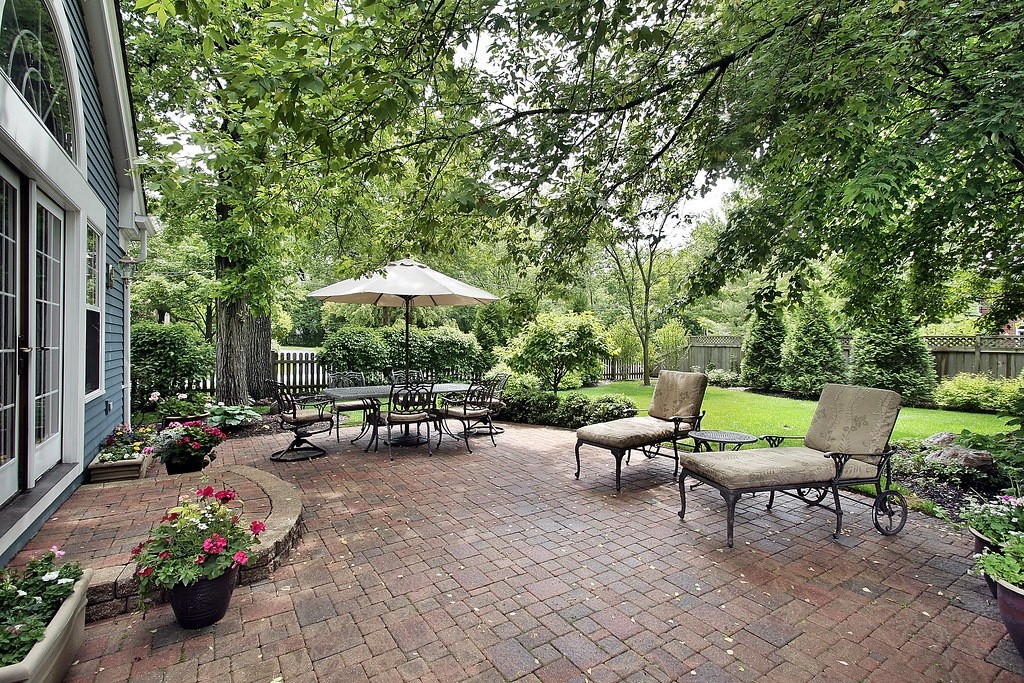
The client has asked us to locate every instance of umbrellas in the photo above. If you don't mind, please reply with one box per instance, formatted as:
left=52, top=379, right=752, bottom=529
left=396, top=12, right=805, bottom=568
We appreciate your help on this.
left=306, top=254, right=501, bottom=447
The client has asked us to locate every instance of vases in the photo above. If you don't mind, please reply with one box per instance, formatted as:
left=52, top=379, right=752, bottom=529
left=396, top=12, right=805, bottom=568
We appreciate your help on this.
left=166, top=411, right=209, bottom=426
left=0, top=569, right=94, bottom=683
left=165, top=564, right=241, bottom=628
left=165, top=455, right=204, bottom=475
left=89, top=448, right=154, bottom=483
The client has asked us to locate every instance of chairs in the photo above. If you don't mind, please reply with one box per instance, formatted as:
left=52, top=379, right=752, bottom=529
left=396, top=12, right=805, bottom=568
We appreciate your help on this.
left=677, top=385, right=908, bottom=546
left=369, top=382, right=436, bottom=457
left=437, top=379, right=497, bottom=453
left=464, top=372, right=514, bottom=434
left=391, top=368, right=433, bottom=432
left=327, top=371, right=381, bottom=441
left=574, top=370, right=709, bottom=494
left=265, top=378, right=333, bottom=462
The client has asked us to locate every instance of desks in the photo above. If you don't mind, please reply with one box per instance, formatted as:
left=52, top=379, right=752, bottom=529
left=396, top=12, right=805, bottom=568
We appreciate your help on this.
left=688, top=429, right=759, bottom=498
left=324, top=384, right=482, bottom=449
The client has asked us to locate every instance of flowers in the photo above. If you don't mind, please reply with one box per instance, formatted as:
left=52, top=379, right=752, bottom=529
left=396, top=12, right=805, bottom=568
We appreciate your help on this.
left=184, top=420, right=226, bottom=446
left=149, top=423, right=207, bottom=464
left=0, top=544, right=85, bottom=668
left=130, top=471, right=265, bottom=621
left=149, top=389, right=211, bottom=417
left=98, top=423, right=153, bottom=463
